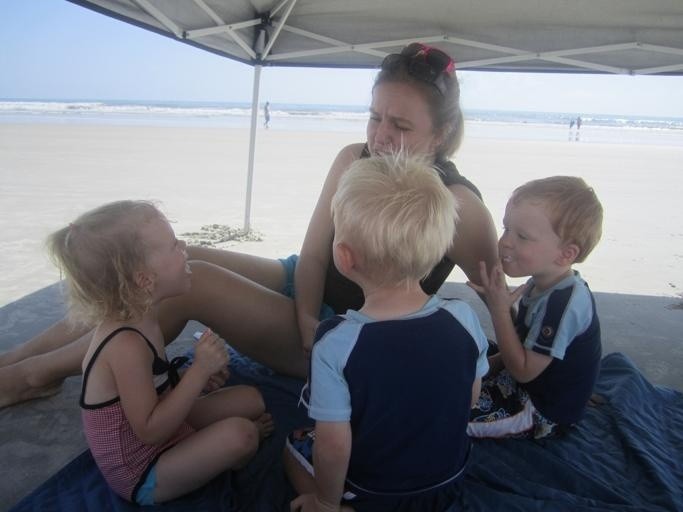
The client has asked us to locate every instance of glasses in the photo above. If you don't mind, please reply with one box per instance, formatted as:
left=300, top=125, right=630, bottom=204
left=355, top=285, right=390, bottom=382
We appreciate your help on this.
left=402, top=43, right=455, bottom=77
left=381, top=54, right=447, bottom=97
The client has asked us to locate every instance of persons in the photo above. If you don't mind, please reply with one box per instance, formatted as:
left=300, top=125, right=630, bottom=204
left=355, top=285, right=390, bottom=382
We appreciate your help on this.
left=464, top=175, right=606, bottom=442
left=280, top=154, right=493, bottom=511
left=0, top=42, right=606, bottom=411
left=36, top=197, right=276, bottom=509
left=568, top=119, right=575, bottom=128
left=261, top=99, right=271, bottom=128
left=575, top=116, right=582, bottom=129
